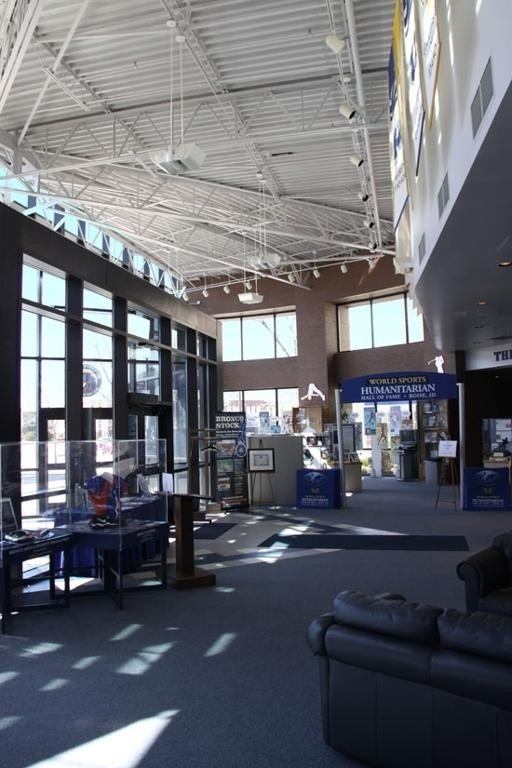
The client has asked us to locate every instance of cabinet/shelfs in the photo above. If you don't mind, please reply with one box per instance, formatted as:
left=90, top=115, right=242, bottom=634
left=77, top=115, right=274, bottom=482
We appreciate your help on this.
left=292, top=405, right=329, bottom=433
left=332, top=461, right=363, bottom=493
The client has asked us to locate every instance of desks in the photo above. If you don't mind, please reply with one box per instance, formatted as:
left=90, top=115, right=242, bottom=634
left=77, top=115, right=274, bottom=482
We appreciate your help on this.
left=0, top=495, right=170, bottom=634
left=248, top=435, right=303, bottom=504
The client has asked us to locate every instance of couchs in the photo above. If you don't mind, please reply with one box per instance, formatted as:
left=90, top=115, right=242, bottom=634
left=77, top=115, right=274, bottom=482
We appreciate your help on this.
left=305, top=528, right=512, bottom=768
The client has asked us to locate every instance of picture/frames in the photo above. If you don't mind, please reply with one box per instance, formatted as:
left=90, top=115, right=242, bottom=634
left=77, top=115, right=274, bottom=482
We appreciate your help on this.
left=247, top=448, right=275, bottom=473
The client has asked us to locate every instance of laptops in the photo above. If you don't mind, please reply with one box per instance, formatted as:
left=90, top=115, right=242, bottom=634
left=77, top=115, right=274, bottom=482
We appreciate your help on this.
left=0, top=497, right=35, bottom=551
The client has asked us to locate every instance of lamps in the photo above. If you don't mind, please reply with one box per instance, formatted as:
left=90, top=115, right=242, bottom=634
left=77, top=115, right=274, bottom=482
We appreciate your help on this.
left=313, top=269, right=320, bottom=279
left=358, top=191, right=369, bottom=202
left=368, top=258, right=376, bottom=268
left=363, top=220, right=374, bottom=229
left=340, top=264, right=348, bottom=274
left=247, top=172, right=279, bottom=272
left=223, top=285, right=231, bottom=295
left=350, top=154, right=364, bottom=167
left=153, top=21, right=205, bottom=177
left=338, top=105, right=355, bottom=120
left=288, top=274, right=295, bottom=282
left=237, top=227, right=264, bottom=305
left=325, top=35, right=345, bottom=53
left=367, top=241, right=377, bottom=251
left=202, top=288, right=209, bottom=297
left=246, top=281, right=253, bottom=289
left=182, top=292, right=189, bottom=302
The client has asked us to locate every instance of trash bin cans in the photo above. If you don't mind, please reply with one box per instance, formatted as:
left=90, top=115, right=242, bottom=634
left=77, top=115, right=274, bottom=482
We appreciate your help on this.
left=423, top=459, right=438, bottom=489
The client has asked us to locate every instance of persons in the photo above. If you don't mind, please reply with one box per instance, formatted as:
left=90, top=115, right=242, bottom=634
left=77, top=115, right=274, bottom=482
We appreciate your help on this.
left=365, top=409, right=375, bottom=427
left=378, top=425, right=387, bottom=446
